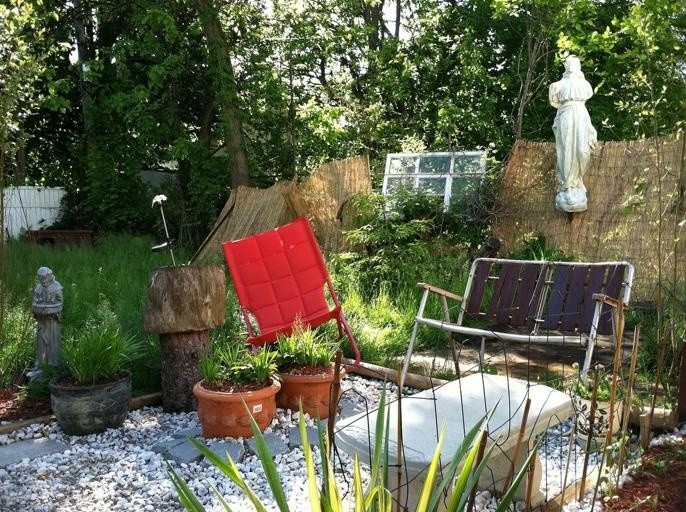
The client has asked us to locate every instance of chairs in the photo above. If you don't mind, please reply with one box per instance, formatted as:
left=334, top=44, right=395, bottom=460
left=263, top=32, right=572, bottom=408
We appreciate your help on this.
left=222, top=213, right=362, bottom=373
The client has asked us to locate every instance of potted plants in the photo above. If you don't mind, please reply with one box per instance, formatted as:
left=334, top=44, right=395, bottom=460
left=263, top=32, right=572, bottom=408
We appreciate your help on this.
left=47, top=320, right=150, bottom=436
left=266, top=311, right=346, bottom=421
left=634, top=375, right=648, bottom=393
left=191, top=334, right=282, bottom=439
left=568, top=360, right=626, bottom=445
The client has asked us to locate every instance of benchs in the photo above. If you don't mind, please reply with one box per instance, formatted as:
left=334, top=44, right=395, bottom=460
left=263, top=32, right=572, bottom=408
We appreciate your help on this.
left=333, top=372, right=575, bottom=512
left=400, top=257, right=635, bottom=394
